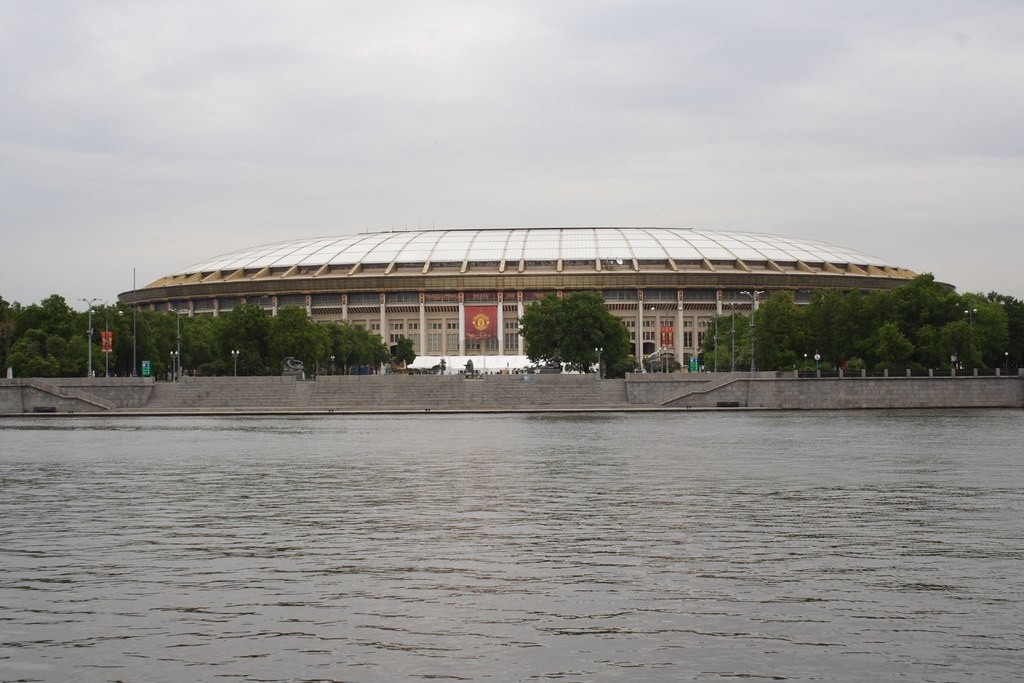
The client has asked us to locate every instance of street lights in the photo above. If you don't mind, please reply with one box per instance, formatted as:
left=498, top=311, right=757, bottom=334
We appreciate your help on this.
left=803, top=353, right=808, bottom=377
left=170, top=350, right=179, bottom=383
left=1005, top=351, right=1009, bottom=376
left=91, top=301, right=124, bottom=376
left=965, top=305, right=979, bottom=328
left=231, top=349, right=240, bottom=377
left=650, top=305, right=683, bottom=373
left=596, top=346, right=604, bottom=381
left=77, top=296, right=99, bottom=377
left=168, top=307, right=189, bottom=383
left=739, top=289, right=766, bottom=374
